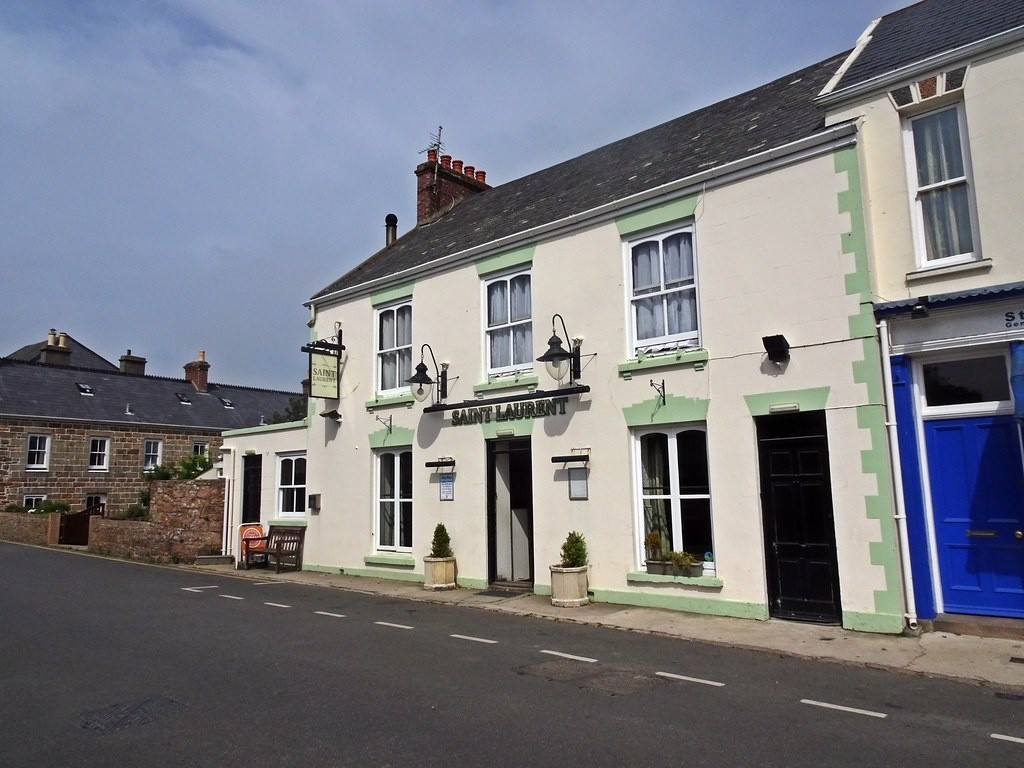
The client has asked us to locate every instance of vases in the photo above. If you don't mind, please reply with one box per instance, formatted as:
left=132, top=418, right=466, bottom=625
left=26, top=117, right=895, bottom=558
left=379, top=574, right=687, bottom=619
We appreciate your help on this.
left=194, top=555, right=234, bottom=565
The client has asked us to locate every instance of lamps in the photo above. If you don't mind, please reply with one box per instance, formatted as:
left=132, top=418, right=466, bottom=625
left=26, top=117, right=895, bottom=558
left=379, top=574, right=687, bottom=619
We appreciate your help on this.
left=405, top=344, right=448, bottom=403
left=762, top=334, right=790, bottom=362
left=911, top=304, right=930, bottom=319
left=537, top=314, right=581, bottom=386
left=319, top=409, right=343, bottom=425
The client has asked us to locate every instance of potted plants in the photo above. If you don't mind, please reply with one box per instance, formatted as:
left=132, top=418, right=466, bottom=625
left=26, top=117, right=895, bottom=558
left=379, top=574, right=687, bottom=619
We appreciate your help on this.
left=423, top=522, right=455, bottom=591
left=549, top=531, right=590, bottom=607
left=643, top=532, right=703, bottom=577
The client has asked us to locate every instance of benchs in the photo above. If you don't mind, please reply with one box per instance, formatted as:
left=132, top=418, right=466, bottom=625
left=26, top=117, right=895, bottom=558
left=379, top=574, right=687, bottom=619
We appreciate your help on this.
left=242, top=525, right=307, bottom=574
